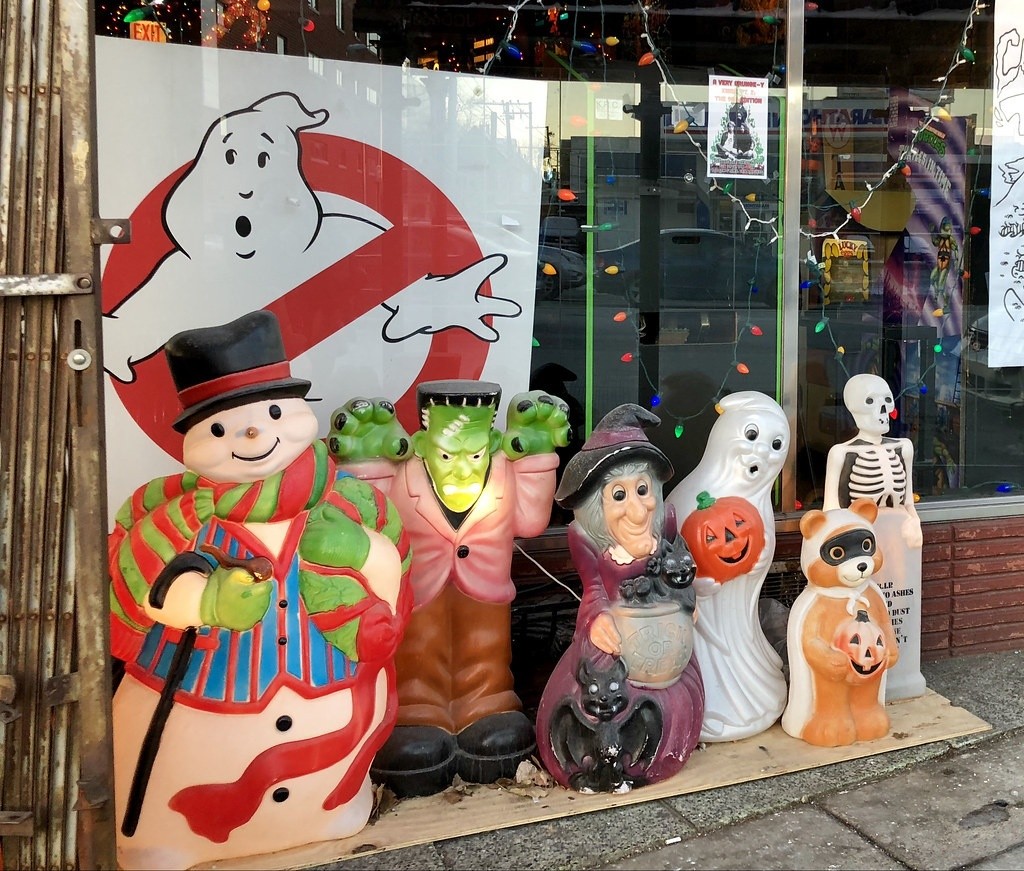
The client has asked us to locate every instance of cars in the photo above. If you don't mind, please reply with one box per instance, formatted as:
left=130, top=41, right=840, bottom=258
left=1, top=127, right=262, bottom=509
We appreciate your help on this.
left=957, top=310, right=1024, bottom=421
left=530, top=227, right=828, bottom=308
